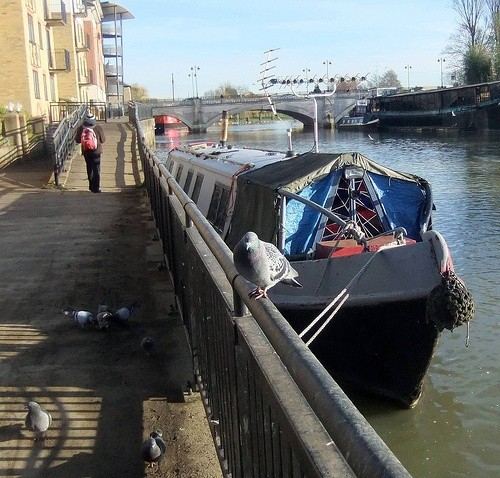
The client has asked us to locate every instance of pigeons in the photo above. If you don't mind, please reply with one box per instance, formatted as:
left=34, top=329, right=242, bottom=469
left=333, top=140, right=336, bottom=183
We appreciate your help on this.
left=141, top=429, right=166, bottom=474
left=234, top=231, right=303, bottom=300
left=25, top=402, right=52, bottom=441
left=56, top=303, right=138, bottom=331
left=127, top=337, right=171, bottom=393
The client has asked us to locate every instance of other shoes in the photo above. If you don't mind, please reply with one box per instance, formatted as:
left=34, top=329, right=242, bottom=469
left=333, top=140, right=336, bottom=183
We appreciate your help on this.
left=93, top=190, right=101, bottom=193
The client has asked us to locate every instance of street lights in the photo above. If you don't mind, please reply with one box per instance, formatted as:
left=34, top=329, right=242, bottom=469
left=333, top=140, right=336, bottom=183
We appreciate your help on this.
left=191, top=65, right=200, bottom=98
left=187, top=72, right=196, bottom=97
left=438, top=56, right=446, bottom=86
left=322, top=60, right=331, bottom=78
left=404, top=65, right=411, bottom=87
left=303, top=67, right=311, bottom=94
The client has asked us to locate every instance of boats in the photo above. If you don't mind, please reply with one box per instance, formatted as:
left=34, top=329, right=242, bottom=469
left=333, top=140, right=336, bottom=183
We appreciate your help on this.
left=164, top=48, right=475, bottom=333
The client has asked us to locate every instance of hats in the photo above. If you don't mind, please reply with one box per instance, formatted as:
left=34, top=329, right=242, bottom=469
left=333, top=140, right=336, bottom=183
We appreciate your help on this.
left=86, top=113, right=95, bottom=120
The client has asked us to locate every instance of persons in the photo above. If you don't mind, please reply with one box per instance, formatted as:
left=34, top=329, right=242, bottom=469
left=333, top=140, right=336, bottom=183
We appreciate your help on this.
left=75, top=110, right=106, bottom=194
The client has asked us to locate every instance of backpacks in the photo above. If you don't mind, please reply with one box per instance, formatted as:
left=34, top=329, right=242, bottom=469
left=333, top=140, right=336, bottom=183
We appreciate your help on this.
left=81, top=124, right=98, bottom=150
